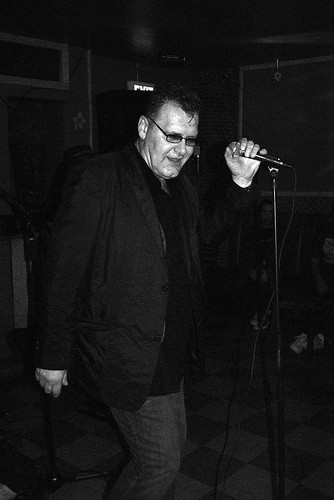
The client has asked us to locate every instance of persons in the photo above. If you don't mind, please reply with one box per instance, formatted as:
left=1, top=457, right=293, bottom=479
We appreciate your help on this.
left=33, top=79, right=268, bottom=500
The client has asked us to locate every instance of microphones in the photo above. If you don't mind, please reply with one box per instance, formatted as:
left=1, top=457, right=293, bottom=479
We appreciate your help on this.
left=228, top=142, right=290, bottom=169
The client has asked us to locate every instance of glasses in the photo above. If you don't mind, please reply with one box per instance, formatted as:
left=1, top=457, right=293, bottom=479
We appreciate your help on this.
left=147, top=115, right=201, bottom=146
